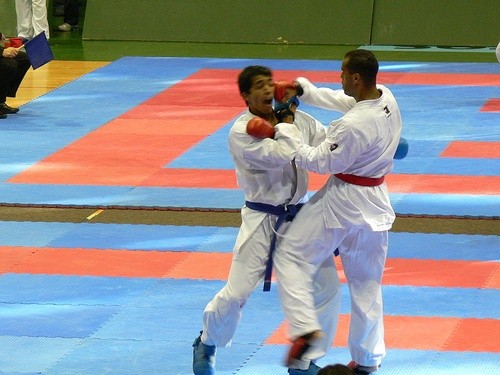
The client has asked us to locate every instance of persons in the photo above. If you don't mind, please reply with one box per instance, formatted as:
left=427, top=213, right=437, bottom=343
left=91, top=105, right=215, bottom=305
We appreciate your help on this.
left=57, top=0, right=79, bottom=32
left=192, top=66, right=408, bottom=375
left=14, top=0, right=50, bottom=46
left=246, top=49, right=402, bottom=375
left=0, top=33, right=31, bottom=119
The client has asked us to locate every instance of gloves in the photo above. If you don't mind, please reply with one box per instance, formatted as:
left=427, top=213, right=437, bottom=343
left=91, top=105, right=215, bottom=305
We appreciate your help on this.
left=273, top=96, right=300, bottom=120
left=393, top=138, right=409, bottom=159
left=246, top=116, right=275, bottom=139
left=274, top=80, right=302, bottom=102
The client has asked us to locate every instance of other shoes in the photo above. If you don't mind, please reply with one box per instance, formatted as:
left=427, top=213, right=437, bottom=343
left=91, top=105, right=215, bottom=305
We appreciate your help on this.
left=58, top=23, right=71, bottom=31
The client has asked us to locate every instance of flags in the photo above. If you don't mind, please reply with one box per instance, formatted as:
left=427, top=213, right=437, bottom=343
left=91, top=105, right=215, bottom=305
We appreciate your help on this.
left=25, top=32, right=54, bottom=70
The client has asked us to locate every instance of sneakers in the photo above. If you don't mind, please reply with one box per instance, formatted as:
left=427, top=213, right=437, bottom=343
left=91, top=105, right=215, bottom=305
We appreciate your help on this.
left=288, top=362, right=322, bottom=375
left=192, top=333, right=217, bottom=374
left=285, top=331, right=328, bottom=368
left=346, top=360, right=377, bottom=375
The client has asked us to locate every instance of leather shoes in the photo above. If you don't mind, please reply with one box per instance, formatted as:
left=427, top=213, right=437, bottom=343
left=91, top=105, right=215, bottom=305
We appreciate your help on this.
left=0, top=114, right=7, bottom=119
left=0, top=103, right=20, bottom=113
